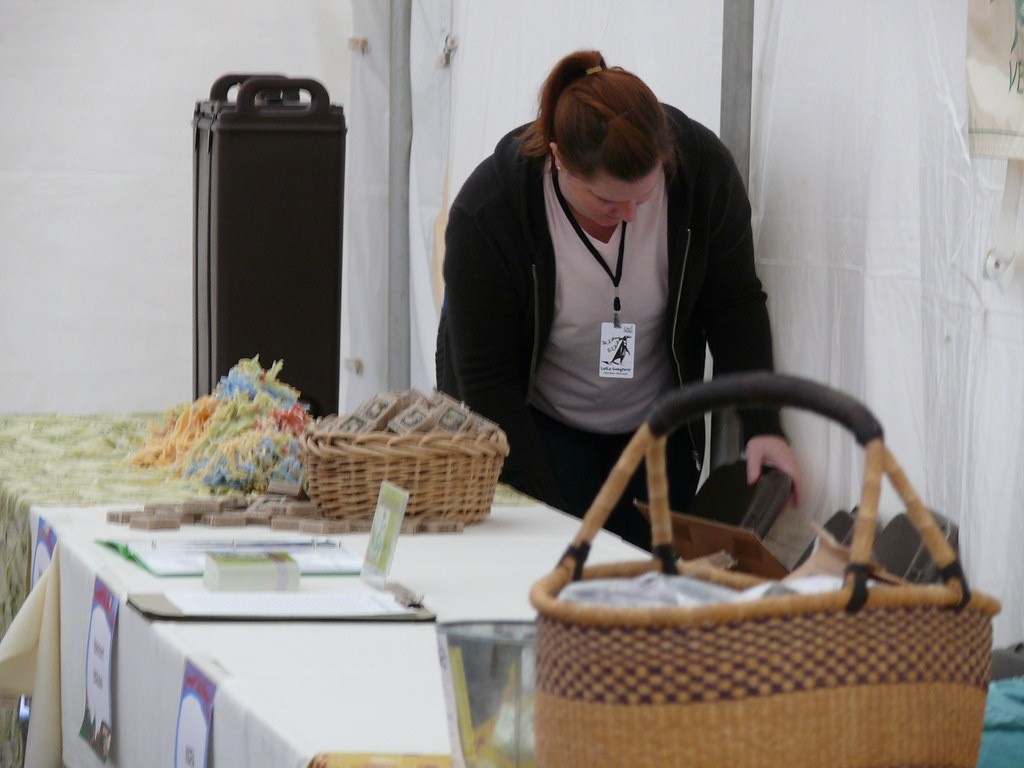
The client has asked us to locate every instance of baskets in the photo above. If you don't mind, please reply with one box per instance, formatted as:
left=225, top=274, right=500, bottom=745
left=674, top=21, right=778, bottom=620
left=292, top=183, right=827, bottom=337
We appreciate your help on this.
left=530, top=374, right=1001, bottom=768
left=300, top=397, right=508, bottom=525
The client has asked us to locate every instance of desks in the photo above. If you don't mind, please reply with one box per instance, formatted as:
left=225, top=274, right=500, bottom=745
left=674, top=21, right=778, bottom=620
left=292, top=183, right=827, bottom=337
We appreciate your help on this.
left=0, top=411, right=1024, bottom=768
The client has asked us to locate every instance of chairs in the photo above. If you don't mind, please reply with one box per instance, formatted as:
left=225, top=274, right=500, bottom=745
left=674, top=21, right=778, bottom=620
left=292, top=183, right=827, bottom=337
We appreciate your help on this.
left=794, top=505, right=883, bottom=568
left=873, top=510, right=958, bottom=583
left=685, top=458, right=794, bottom=539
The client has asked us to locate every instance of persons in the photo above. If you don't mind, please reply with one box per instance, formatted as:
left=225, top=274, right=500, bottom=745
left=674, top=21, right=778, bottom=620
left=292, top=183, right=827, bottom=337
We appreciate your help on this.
left=435, top=50, right=802, bottom=552
